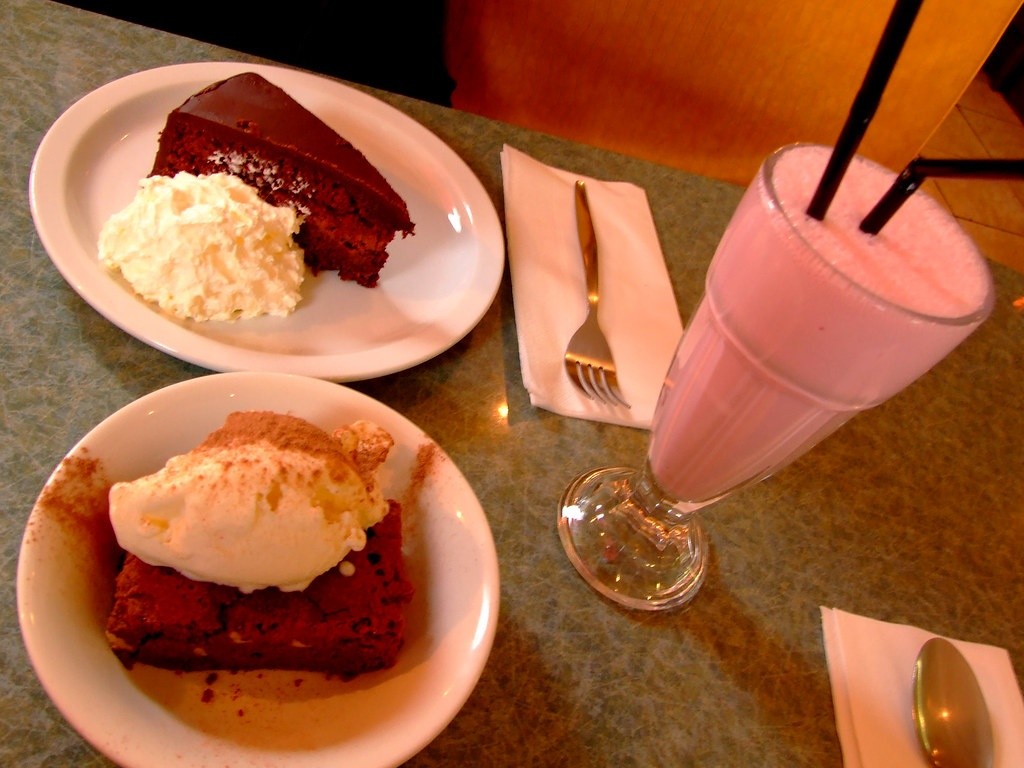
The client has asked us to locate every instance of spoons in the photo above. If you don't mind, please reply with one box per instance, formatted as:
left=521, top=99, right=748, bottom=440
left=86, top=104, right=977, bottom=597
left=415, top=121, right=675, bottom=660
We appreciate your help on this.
left=912, top=637, right=995, bottom=768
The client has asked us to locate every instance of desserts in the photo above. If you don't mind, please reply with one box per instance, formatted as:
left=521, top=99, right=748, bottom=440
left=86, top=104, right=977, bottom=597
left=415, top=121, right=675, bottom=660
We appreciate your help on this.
left=106, top=411, right=416, bottom=676
left=97, top=70, right=415, bottom=322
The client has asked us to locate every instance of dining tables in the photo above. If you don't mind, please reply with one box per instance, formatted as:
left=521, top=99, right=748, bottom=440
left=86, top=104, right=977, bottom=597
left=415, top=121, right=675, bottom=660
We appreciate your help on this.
left=1, top=0, right=1024, bottom=768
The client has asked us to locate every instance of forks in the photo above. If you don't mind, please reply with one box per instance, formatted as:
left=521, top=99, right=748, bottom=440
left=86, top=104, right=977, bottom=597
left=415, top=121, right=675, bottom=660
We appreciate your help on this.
left=565, top=180, right=632, bottom=409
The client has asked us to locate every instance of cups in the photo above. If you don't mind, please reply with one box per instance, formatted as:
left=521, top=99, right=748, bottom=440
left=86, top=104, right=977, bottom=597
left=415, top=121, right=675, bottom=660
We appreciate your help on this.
left=557, top=140, right=994, bottom=609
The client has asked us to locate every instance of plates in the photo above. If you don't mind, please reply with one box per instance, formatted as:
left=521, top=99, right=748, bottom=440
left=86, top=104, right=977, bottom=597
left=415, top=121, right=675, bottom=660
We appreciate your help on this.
left=17, top=372, right=498, bottom=768
left=27, top=61, right=505, bottom=384
